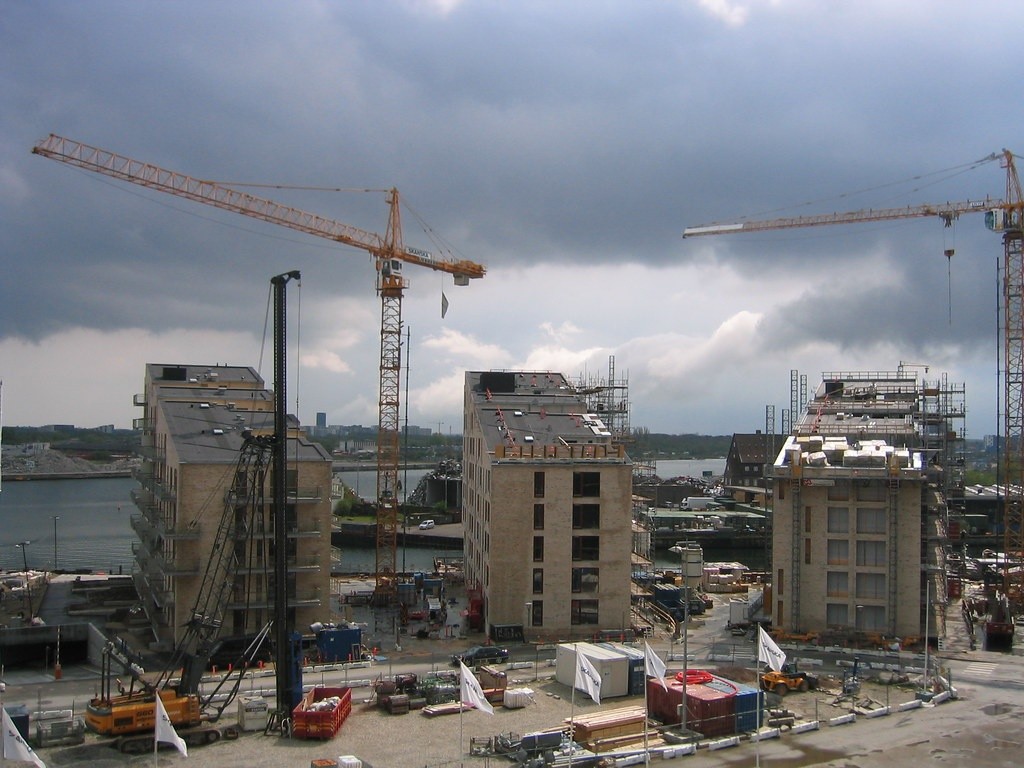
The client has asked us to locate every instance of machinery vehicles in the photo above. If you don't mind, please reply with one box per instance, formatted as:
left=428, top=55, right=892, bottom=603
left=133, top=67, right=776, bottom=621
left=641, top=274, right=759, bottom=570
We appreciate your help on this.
left=81, top=430, right=276, bottom=756
left=761, top=661, right=811, bottom=697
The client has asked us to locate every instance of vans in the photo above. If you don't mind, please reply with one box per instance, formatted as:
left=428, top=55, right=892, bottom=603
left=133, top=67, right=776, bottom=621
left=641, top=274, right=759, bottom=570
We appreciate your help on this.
left=419, top=520, right=434, bottom=530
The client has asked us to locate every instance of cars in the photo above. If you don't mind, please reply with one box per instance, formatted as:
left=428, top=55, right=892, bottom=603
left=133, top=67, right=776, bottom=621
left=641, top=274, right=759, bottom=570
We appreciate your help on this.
left=451, top=645, right=512, bottom=665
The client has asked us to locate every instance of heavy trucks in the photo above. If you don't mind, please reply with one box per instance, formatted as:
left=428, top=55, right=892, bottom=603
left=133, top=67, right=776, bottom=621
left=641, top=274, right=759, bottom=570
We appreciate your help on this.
left=680, top=497, right=714, bottom=512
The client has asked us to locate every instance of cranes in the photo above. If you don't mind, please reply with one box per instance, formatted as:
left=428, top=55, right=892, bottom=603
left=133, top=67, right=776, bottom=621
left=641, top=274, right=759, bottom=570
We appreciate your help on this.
left=683, top=146, right=1024, bottom=622
left=32, top=134, right=487, bottom=601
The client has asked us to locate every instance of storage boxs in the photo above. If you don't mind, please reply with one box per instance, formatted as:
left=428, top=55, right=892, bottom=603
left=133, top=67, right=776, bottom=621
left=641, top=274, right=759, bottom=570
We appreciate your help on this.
left=293, top=686, right=352, bottom=739
left=647, top=672, right=764, bottom=739
left=555, top=641, right=652, bottom=700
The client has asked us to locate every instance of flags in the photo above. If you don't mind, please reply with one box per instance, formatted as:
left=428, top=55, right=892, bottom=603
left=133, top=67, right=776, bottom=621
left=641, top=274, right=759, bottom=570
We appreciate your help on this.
left=2, top=708, right=46, bottom=768
left=645, top=641, right=668, bottom=692
left=574, top=646, right=601, bottom=705
left=759, top=626, right=786, bottom=673
left=157, top=695, right=189, bottom=757
left=461, top=661, right=494, bottom=715
